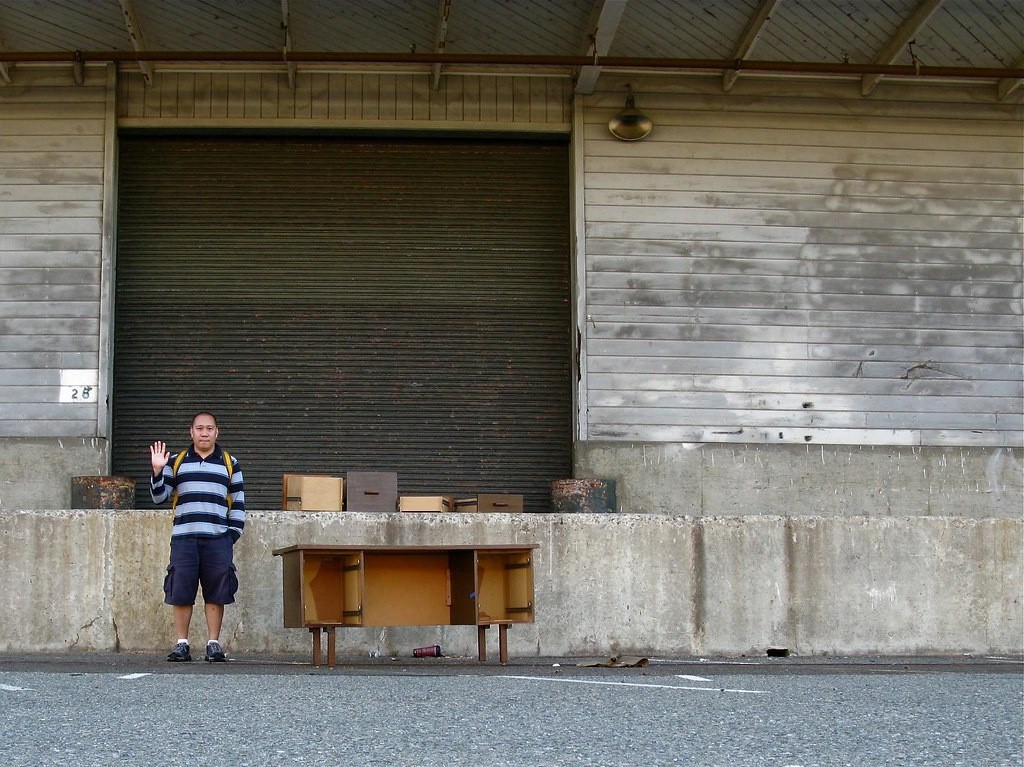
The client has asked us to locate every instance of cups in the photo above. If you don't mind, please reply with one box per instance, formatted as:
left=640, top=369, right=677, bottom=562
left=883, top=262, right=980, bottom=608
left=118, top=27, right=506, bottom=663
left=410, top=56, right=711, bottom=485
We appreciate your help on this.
left=412, top=645, right=440, bottom=657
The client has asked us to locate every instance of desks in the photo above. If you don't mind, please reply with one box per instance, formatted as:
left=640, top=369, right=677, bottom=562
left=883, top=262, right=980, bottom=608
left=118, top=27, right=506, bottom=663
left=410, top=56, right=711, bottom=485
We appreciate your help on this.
left=272, top=544, right=540, bottom=668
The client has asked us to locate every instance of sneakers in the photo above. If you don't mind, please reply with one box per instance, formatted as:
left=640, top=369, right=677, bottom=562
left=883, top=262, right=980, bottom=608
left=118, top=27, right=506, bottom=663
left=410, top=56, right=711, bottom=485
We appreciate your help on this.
left=204, top=642, right=225, bottom=662
left=167, top=642, right=191, bottom=662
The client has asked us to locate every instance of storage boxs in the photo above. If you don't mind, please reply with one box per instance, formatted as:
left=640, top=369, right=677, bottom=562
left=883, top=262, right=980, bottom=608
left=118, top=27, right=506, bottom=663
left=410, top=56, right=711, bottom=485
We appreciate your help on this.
left=283, top=472, right=524, bottom=513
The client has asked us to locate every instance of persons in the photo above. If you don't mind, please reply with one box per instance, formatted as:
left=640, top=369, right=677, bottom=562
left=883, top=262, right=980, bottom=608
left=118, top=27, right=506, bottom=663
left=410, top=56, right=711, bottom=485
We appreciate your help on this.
left=150, top=411, right=246, bottom=662
